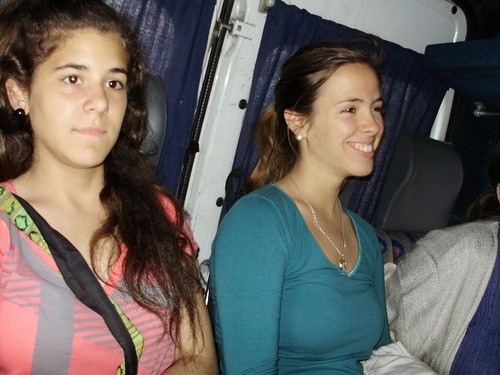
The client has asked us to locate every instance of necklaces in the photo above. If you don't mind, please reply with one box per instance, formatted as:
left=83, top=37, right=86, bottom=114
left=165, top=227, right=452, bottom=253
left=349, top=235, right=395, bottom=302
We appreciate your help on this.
left=286, top=170, right=347, bottom=272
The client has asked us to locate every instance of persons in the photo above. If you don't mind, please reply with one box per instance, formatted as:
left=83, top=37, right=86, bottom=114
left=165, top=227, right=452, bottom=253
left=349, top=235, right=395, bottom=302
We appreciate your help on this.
left=0, top=0, right=220, bottom=375
left=360, top=181, right=500, bottom=375
left=207, top=43, right=393, bottom=375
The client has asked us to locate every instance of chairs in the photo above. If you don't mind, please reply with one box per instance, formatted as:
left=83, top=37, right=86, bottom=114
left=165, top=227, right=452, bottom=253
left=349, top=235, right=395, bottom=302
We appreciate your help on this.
left=378, top=132, right=465, bottom=265
